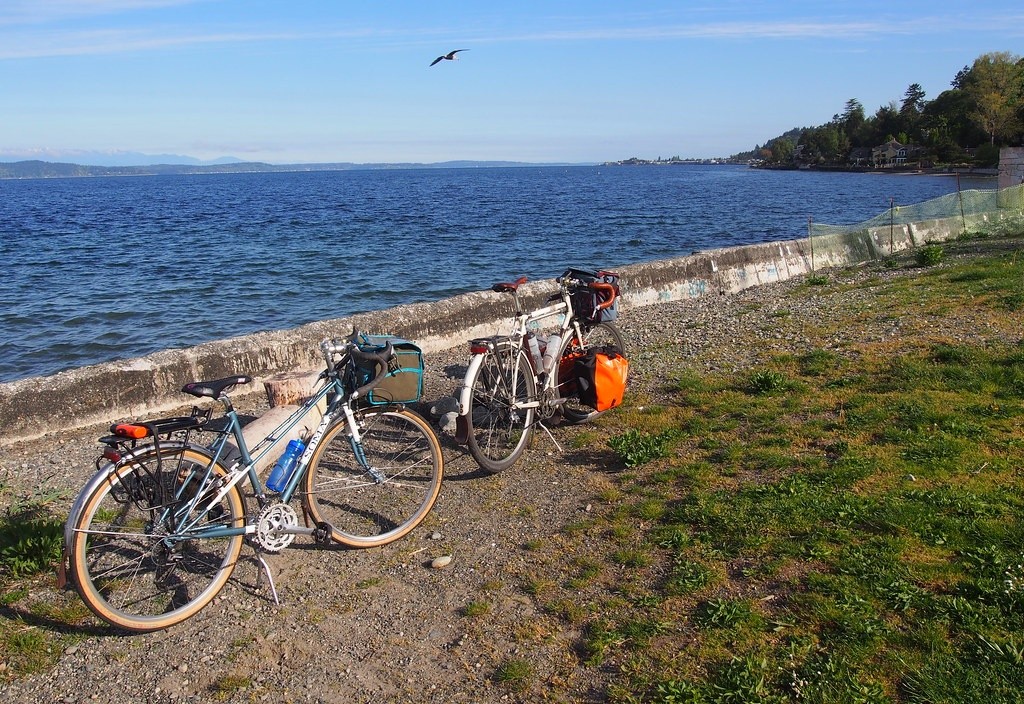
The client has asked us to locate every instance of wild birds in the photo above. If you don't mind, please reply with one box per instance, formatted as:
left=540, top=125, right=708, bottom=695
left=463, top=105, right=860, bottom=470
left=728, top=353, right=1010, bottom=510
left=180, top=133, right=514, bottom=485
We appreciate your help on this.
left=430, top=49, right=469, bottom=66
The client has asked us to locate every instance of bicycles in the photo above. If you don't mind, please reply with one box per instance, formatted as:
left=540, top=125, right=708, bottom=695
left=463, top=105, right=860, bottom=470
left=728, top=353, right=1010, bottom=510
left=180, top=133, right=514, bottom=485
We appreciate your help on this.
left=460, top=271, right=629, bottom=479
left=59, top=319, right=447, bottom=636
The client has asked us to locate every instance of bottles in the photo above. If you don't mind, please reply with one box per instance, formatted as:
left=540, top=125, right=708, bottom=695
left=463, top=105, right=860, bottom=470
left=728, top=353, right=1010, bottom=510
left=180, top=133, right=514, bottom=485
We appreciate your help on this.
left=544, top=333, right=562, bottom=372
left=266, top=439, right=306, bottom=493
left=528, top=332, right=543, bottom=372
